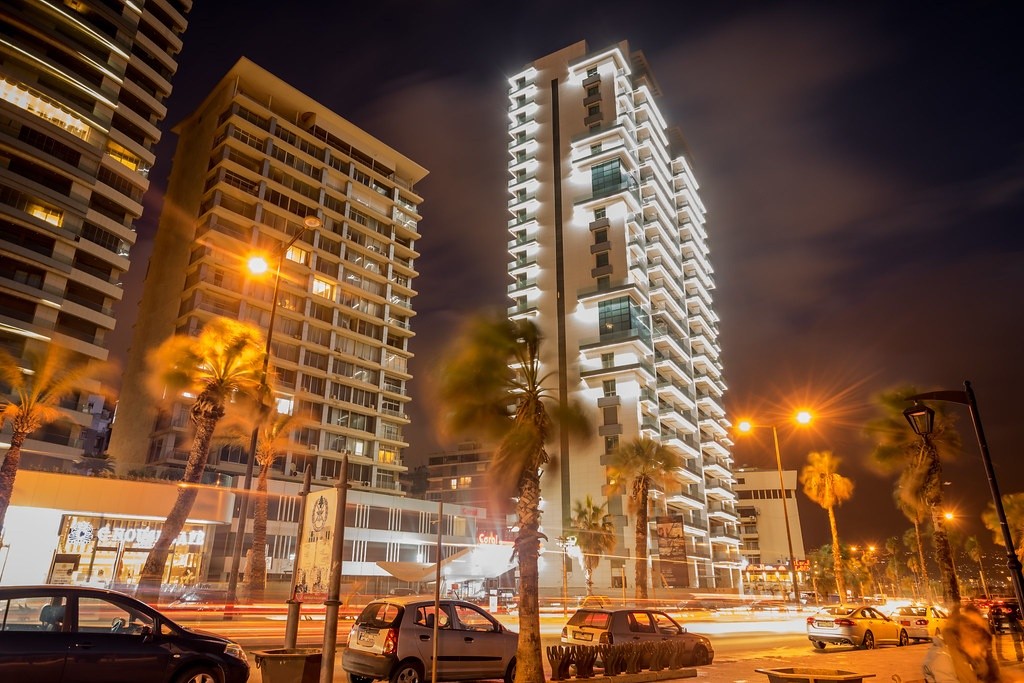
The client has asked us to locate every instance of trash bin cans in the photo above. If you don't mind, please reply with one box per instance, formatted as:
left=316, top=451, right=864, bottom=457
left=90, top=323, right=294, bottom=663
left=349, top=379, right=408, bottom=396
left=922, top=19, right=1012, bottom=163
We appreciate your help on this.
left=252, top=647, right=338, bottom=683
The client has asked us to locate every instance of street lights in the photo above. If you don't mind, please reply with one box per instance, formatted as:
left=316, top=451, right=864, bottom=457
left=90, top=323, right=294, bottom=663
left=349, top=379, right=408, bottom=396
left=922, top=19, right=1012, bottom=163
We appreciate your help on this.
left=903, top=379, right=1024, bottom=619
left=223, top=215, right=322, bottom=623
left=737, top=409, right=814, bottom=613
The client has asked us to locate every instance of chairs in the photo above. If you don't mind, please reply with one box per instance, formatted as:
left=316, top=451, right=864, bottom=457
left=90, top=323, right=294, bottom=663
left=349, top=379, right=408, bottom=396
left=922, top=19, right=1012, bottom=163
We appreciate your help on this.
left=427, top=613, right=434, bottom=628
left=416, top=610, right=423, bottom=625
left=34, top=605, right=65, bottom=631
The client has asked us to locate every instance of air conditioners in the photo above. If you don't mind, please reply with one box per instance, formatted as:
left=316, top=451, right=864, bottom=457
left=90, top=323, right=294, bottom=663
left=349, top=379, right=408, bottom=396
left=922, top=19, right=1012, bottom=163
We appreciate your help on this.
left=749, top=516, right=755, bottom=520
left=368, top=403, right=374, bottom=410
left=292, top=471, right=301, bottom=479
left=405, top=415, right=408, bottom=419
left=392, top=460, right=398, bottom=466
left=341, top=449, right=346, bottom=454
left=323, top=476, right=329, bottom=481
left=776, top=559, right=782, bottom=564
left=300, top=387, right=307, bottom=393
left=330, top=394, right=336, bottom=400
left=310, top=444, right=317, bottom=451
left=336, top=347, right=342, bottom=352
left=363, top=481, right=371, bottom=487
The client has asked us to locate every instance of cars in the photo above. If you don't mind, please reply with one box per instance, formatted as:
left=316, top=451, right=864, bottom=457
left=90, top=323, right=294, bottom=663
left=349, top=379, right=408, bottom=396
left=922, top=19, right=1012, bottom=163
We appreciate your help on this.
left=169, top=587, right=242, bottom=612
left=988, top=600, right=1024, bottom=635
left=391, top=588, right=420, bottom=598
left=341, top=593, right=519, bottom=683
left=886, top=605, right=950, bottom=643
left=807, top=603, right=909, bottom=650
left=1, top=583, right=251, bottom=683
left=559, top=605, right=714, bottom=672
